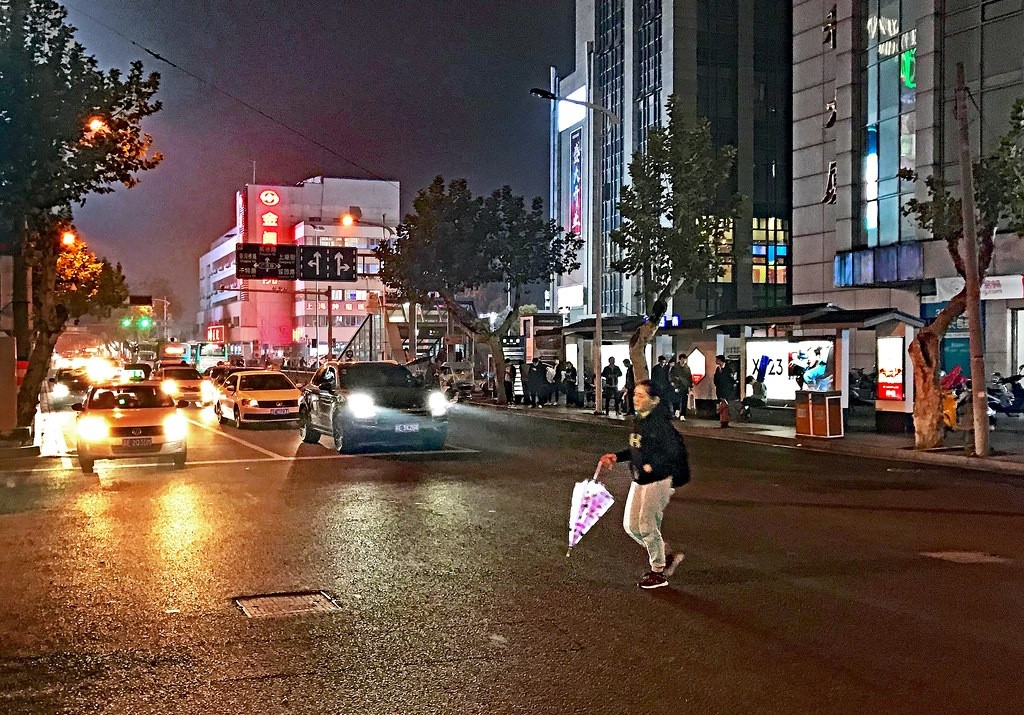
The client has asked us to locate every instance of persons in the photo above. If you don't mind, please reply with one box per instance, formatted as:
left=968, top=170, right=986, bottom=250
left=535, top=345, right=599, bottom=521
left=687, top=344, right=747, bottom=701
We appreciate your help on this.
left=133, top=388, right=154, bottom=408
left=712, top=355, right=735, bottom=421
left=237, top=351, right=307, bottom=369
left=442, top=354, right=695, bottom=422
left=786, top=348, right=833, bottom=392
left=599, top=380, right=685, bottom=589
left=739, top=375, right=768, bottom=422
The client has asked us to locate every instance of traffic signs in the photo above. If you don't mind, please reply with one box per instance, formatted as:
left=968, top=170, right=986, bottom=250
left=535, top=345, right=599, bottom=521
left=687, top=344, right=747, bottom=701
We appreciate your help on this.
left=235, top=244, right=299, bottom=280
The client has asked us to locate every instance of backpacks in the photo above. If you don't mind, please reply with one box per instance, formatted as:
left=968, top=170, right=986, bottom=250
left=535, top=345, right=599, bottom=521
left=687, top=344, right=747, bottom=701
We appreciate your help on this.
left=671, top=425, right=692, bottom=488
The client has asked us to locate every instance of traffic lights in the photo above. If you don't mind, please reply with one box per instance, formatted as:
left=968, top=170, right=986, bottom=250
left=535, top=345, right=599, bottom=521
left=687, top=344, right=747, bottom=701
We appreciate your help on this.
left=137, top=318, right=151, bottom=330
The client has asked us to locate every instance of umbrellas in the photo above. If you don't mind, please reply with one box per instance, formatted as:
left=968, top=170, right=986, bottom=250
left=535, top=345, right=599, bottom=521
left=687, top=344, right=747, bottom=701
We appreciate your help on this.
left=566, top=458, right=615, bottom=558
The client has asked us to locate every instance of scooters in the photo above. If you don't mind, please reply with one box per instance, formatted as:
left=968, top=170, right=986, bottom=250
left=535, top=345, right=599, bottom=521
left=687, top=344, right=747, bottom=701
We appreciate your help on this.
left=845, top=365, right=878, bottom=414
left=438, top=361, right=475, bottom=404
left=939, top=365, right=1024, bottom=438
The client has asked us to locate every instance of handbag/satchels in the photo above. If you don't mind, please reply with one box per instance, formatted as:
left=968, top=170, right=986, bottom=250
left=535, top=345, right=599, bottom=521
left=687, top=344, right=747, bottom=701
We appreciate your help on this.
left=687, top=391, right=696, bottom=410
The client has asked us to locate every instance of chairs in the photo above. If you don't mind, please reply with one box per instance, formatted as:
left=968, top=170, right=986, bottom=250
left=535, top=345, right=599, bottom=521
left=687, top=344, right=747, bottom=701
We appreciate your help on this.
left=100, top=391, right=115, bottom=409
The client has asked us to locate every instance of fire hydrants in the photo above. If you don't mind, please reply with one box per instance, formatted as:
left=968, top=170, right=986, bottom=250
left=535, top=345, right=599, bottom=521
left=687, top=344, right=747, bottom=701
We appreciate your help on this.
left=716, top=397, right=732, bottom=428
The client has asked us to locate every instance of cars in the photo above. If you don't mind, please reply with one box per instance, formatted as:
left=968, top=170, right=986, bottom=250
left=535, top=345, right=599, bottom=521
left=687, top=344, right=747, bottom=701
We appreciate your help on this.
left=47, top=355, right=206, bottom=473
left=298, top=360, right=450, bottom=454
left=201, top=365, right=303, bottom=429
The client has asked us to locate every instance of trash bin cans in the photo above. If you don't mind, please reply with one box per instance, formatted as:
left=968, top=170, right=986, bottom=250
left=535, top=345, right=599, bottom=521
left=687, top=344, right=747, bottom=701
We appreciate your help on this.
left=793, top=390, right=845, bottom=439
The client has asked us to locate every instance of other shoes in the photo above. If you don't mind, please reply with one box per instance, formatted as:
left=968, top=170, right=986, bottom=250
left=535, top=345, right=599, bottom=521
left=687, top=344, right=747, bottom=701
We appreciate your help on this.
left=508, top=404, right=517, bottom=409
left=549, top=402, right=552, bottom=405
left=680, top=416, right=686, bottom=422
left=720, top=421, right=731, bottom=428
left=675, top=410, right=680, bottom=418
left=544, top=402, right=549, bottom=405
left=663, top=551, right=685, bottom=578
left=553, top=402, right=557, bottom=405
left=538, top=405, right=542, bottom=409
left=623, top=412, right=635, bottom=416
left=637, top=573, right=669, bottom=589
left=528, top=405, right=533, bottom=408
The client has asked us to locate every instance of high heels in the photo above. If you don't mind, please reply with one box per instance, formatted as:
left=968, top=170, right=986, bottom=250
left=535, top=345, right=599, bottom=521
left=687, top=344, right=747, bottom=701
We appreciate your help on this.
left=616, top=410, right=621, bottom=415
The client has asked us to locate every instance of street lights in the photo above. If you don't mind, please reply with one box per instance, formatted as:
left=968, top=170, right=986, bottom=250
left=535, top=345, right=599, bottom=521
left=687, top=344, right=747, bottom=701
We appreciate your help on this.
left=343, top=215, right=417, bottom=358
left=527, top=86, right=656, bottom=415
left=124, top=319, right=131, bottom=326
